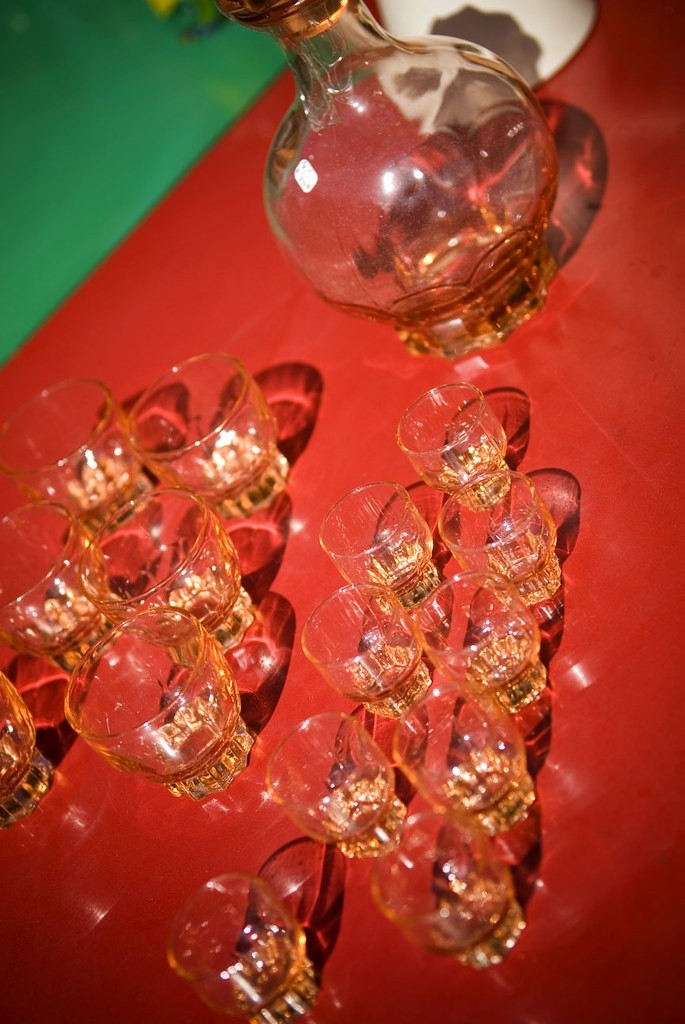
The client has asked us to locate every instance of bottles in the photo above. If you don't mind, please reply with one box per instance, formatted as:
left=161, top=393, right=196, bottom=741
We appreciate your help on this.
left=216, top=0, right=560, bottom=359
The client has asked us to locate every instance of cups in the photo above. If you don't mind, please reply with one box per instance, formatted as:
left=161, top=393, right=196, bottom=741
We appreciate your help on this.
left=395, top=382, right=512, bottom=512
left=265, top=711, right=407, bottom=858
left=374, top=813, right=525, bottom=973
left=165, top=872, right=319, bottom=1024
left=125, top=352, right=290, bottom=521
left=0, top=671, right=55, bottom=829
left=436, top=471, right=564, bottom=605
left=391, top=681, right=538, bottom=837
left=62, top=606, right=254, bottom=801
left=412, top=570, right=555, bottom=746
left=300, top=581, right=432, bottom=719
left=319, top=482, right=440, bottom=614
left=79, top=487, right=256, bottom=664
left=0, top=500, right=118, bottom=673
left=0, top=377, right=155, bottom=537
